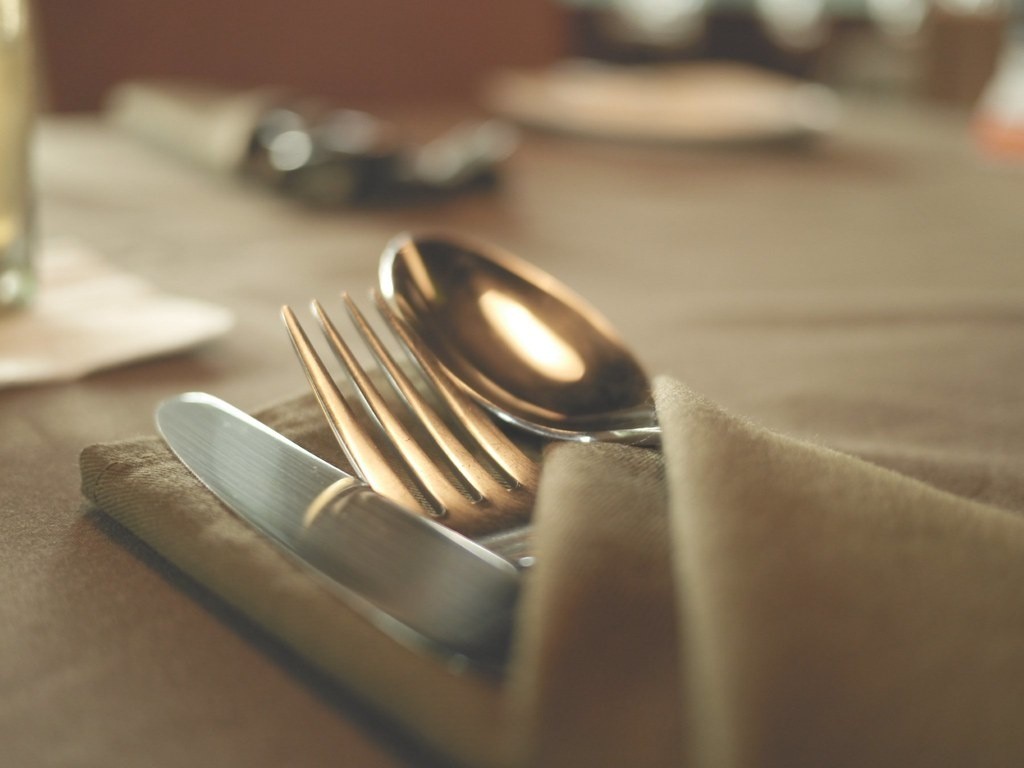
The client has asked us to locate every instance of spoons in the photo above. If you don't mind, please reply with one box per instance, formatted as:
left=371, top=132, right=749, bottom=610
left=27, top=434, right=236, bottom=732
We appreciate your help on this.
left=379, top=229, right=660, bottom=443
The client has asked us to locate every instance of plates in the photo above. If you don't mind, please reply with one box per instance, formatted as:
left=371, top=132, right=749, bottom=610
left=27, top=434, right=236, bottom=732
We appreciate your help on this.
left=484, top=63, right=841, bottom=145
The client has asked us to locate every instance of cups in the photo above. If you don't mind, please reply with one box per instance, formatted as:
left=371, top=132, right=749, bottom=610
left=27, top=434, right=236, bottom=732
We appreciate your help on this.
left=0, top=0, right=34, bottom=309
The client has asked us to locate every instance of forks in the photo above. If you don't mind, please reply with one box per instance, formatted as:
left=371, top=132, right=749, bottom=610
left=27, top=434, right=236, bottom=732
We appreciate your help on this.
left=283, top=286, right=537, bottom=567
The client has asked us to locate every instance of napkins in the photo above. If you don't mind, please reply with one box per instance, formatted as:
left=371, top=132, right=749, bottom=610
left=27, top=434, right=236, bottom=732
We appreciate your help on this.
left=76, top=357, right=1024, bottom=768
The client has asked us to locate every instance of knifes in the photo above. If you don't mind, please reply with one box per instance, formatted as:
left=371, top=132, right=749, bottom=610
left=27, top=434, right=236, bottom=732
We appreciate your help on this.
left=155, top=392, right=516, bottom=663
